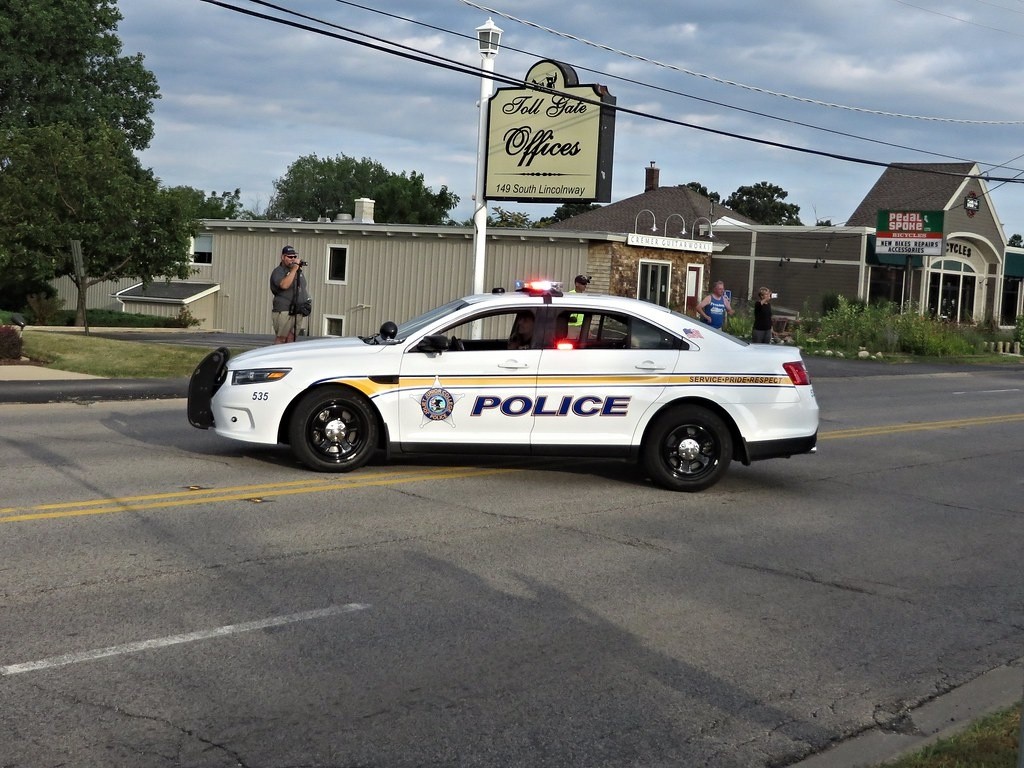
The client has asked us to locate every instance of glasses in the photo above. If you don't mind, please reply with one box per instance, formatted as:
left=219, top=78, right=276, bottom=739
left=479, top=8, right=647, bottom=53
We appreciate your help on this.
left=284, top=255, right=297, bottom=259
left=581, top=283, right=587, bottom=285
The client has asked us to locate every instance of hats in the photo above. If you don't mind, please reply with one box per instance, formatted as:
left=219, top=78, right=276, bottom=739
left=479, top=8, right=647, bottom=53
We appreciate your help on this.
left=575, top=274, right=590, bottom=283
left=282, top=245, right=299, bottom=254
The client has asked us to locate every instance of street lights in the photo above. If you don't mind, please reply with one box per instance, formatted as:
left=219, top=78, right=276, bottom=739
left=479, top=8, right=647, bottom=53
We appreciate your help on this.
left=472, top=10, right=502, bottom=339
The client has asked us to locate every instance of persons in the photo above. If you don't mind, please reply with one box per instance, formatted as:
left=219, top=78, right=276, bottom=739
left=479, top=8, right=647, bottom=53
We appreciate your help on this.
left=696, top=281, right=735, bottom=331
left=269, top=246, right=307, bottom=344
left=508, top=312, right=535, bottom=350
left=568, top=275, right=593, bottom=339
left=753, top=287, right=773, bottom=344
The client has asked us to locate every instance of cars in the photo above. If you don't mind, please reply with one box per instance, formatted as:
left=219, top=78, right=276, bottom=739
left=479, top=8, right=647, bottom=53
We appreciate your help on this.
left=186, top=282, right=819, bottom=492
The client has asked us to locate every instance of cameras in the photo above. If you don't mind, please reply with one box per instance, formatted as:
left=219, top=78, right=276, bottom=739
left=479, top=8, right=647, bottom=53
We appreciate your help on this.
left=299, top=259, right=308, bottom=268
left=771, top=293, right=777, bottom=298
